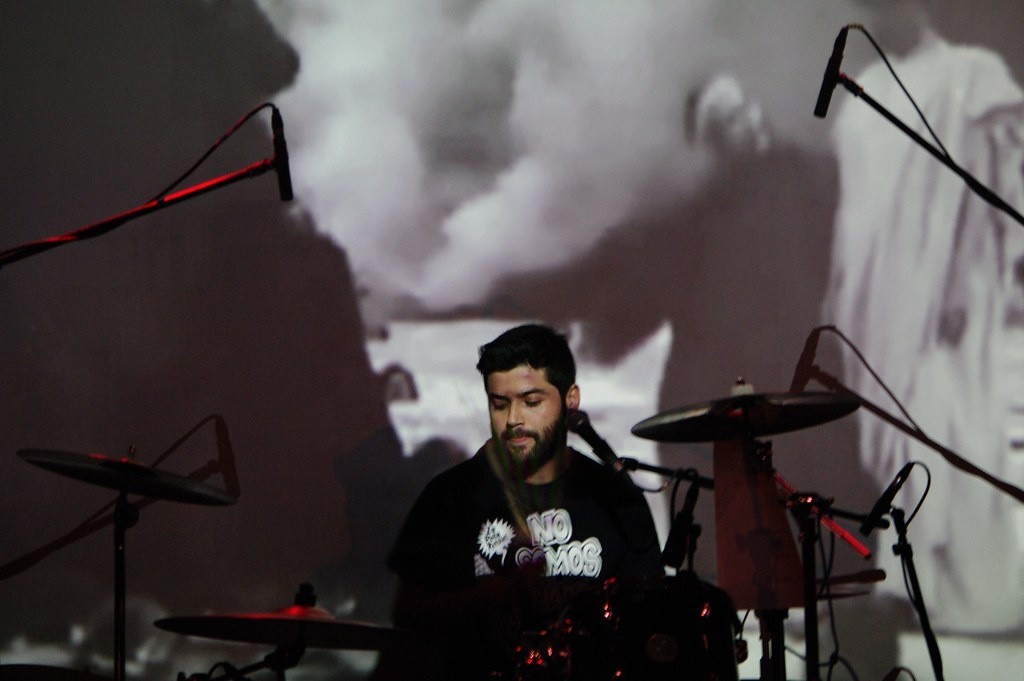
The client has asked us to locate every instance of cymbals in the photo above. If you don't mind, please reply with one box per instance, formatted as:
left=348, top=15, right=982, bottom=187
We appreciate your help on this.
left=153, top=607, right=391, bottom=649
left=631, top=392, right=862, bottom=444
left=18, top=448, right=239, bottom=506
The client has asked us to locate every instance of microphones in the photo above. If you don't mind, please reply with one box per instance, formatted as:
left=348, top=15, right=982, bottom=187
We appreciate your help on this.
left=271, top=104, right=294, bottom=202
left=814, top=26, right=850, bottom=119
left=661, top=483, right=700, bottom=568
left=859, top=463, right=916, bottom=536
left=562, top=408, right=632, bottom=486
left=213, top=416, right=241, bottom=500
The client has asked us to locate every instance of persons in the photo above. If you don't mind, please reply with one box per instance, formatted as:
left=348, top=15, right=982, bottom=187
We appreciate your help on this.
left=365, top=324, right=662, bottom=681
left=477, top=0, right=1024, bottom=645
